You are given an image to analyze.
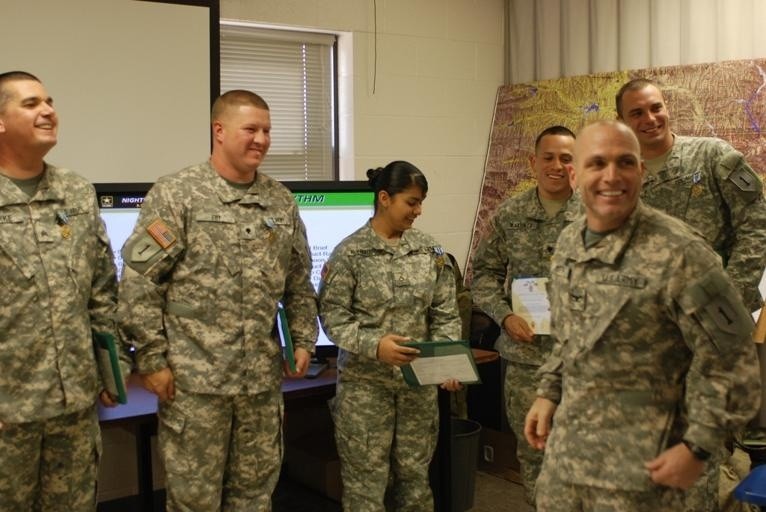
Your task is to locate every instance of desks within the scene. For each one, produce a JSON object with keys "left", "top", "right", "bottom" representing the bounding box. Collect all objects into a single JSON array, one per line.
[{"left": 96, "top": 345, "right": 501, "bottom": 511}]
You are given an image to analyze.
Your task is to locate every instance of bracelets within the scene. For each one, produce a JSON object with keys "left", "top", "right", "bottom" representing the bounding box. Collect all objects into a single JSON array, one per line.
[{"left": 683, "top": 440, "right": 710, "bottom": 463}]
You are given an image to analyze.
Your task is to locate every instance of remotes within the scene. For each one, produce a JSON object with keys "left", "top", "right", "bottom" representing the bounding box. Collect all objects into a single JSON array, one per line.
[{"left": 305, "top": 363, "right": 328, "bottom": 378}]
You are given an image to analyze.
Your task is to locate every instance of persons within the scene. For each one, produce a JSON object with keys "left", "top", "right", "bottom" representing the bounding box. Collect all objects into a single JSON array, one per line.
[
  {"left": 615, "top": 76, "right": 765, "bottom": 512},
  {"left": 0, "top": 70, "right": 133, "bottom": 512},
  {"left": 317, "top": 157, "right": 468, "bottom": 511},
  {"left": 114, "top": 89, "right": 319, "bottom": 512},
  {"left": 468, "top": 124, "right": 585, "bottom": 511},
  {"left": 522, "top": 116, "right": 766, "bottom": 512}
]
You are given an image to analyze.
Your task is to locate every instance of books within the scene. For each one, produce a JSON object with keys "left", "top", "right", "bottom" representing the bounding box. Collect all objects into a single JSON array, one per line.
[
  {"left": 90, "top": 330, "right": 127, "bottom": 406},
  {"left": 277, "top": 306, "right": 297, "bottom": 376},
  {"left": 397, "top": 340, "right": 483, "bottom": 392}
]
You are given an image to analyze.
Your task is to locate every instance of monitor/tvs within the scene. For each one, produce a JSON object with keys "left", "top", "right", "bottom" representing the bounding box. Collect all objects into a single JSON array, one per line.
[
  {"left": 277, "top": 181, "right": 375, "bottom": 369},
  {"left": 92, "top": 182, "right": 154, "bottom": 373}
]
